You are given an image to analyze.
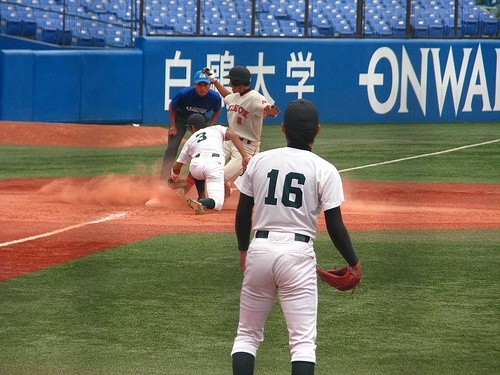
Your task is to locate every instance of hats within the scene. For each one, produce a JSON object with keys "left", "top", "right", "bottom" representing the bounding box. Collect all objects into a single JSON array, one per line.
[
  {"left": 284, "top": 98, "right": 318, "bottom": 129},
  {"left": 193, "top": 70, "right": 211, "bottom": 84},
  {"left": 223, "top": 66, "right": 251, "bottom": 83},
  {"left": 185, "top": 113, "right": 206, "bottom": 128}
]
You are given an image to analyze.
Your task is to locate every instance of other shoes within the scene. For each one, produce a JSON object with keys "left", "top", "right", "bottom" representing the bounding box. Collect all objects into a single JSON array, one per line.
[{"left": 186, "top": 198, "right": 204, "bottom": 214}]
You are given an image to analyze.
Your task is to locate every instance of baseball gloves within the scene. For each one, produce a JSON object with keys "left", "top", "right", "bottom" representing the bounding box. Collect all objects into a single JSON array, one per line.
[
  {"left": 318, "top": 266, "right": 360, "bottom": 291},
  {"left": 167, "top": 177, "right": 188, "bottom": 189}
]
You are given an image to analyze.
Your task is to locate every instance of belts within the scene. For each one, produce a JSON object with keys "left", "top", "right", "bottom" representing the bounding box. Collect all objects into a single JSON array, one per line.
[{"left": 255, "top": 230, "right": 311, "bottom": 243}]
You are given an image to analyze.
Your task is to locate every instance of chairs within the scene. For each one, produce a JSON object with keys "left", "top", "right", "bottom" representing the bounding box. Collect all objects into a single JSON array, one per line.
[{"left": 0, "top": 0, "right": 500, "bottom": 46}]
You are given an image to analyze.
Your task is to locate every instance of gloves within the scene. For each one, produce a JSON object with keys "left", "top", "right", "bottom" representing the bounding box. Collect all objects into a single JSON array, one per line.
[{"left": 202, "top": 67, "right": 217, "bottom": 83}]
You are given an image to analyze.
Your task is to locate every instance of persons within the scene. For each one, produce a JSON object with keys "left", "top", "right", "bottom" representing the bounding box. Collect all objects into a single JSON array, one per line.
[
  {"left": 230, "top": 97, "right": 362, "bottom": 375},
  {"left": 170, "top": 113, "right": 251, "bottom": 215},
  {"left": 175, "top": 66, "right": 280, "bottom": 205},
  {"left": 158, "top": 69, "right": 222, "bottom": 181}
]
